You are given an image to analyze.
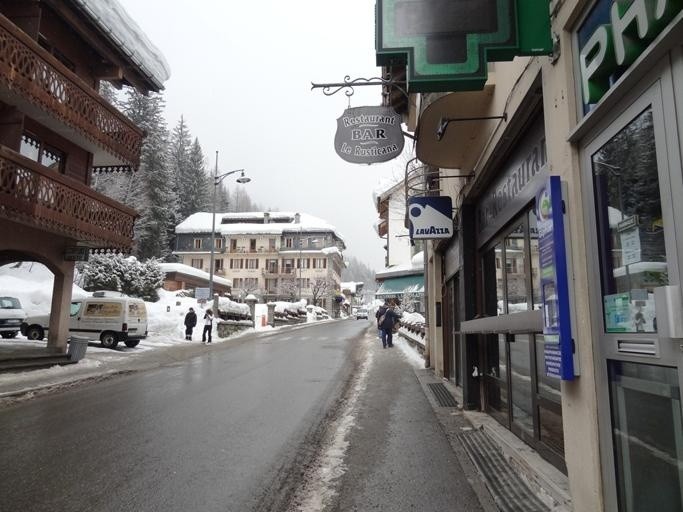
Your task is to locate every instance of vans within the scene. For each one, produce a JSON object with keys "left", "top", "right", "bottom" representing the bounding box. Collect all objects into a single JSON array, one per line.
[
  {"left": 20, "top": 294, "right": 148, "bottom": 349},
  {"left": 0, "top": 295, "right": 27, "bottom": 340}
]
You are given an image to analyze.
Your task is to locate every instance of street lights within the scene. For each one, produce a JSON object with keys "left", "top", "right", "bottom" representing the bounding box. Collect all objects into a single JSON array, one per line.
[{"left": 208, "top": 150, "right": 251, "bottom": 302}]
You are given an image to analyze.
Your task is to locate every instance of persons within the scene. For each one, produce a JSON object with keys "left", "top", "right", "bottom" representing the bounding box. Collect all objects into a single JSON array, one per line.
[
  {"left": 183, "top": 307, "right": 196, "bottom": 340},
  {"left": 201, "top": 308, "right": 214, "bottom": 345},
  {"left": 374, "top": 300, "right": 404, "bottom": 349}
]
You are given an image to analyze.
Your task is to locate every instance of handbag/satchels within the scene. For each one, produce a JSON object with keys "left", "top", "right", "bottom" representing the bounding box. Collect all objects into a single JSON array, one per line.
[{"left": 378, "top": 309, "right": 389, "bottom": 326}]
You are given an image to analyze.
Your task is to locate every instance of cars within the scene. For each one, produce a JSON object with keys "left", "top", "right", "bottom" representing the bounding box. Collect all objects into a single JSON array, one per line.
[{"left": 355, "top": 308, "right": 369, "bottom": 320}]
